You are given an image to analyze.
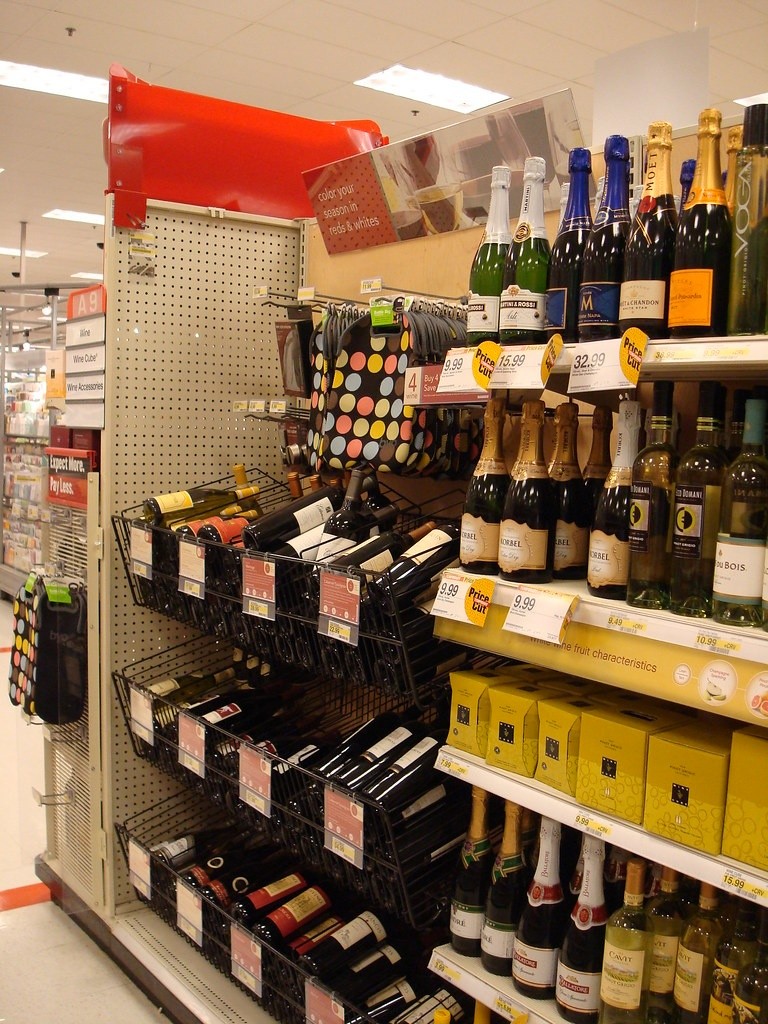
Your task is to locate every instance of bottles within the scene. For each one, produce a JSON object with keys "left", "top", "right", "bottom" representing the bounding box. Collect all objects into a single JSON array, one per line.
[
  {"left": 498, "top": 400, "right": 552, "bottom": 584},
  {"left": 133, "top": 465, "right": 473, "bottom": 695},
  {"left": 461, "top": 398, "right": 511, "bottom": 575},
  {"left": 543, "top": 148, "right": 593, "bottom": 344},
  {"left": 480, "top": 799, "right": 528, "bottom": 976},
  {"left": 136, "top": 817, "right": 471, "bottom": 1024},
  {"left": 558, "top": 103, "right": 768, "bottom": 337},
  {"left": 498, "top": 156, "right": 551, "bottom": 345},
  {"left": 512, "top": 808, "right": 768, "bottom": 1024},
  {"left": 547, "top": 399, "right": 768, "bottom": 632},
  {"left": 451, "top": 784, "right": 495, "bottom": 957},
  {"left": 467, "top": 165, "right": 513, "bottom": 348},
  {"left": 113, "top": 641, "right": 472, "bottom": 933}
]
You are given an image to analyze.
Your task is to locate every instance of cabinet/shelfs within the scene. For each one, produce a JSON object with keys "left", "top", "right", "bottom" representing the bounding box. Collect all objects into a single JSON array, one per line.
[{"left": 427, "top": 322, "right": 768, "bottom": 1024}]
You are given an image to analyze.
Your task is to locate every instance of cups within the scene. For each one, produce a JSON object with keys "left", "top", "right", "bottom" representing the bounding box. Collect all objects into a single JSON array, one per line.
[
  {"left": 372, "top": 131, "right": 463, "bottom": 242},
  {"left": 440, "top": 110, "right": 533, "bottom": 230},
  {"left": 543, "top": 91, "right": 584, "bottom": 176}
]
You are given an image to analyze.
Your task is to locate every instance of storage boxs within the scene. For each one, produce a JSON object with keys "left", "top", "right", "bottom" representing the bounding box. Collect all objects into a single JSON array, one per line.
[
  {"left": 445, "top": 665, "right": 768, "bottom": 875},
  {"left": 49, "top": 425, "right": 100, "bottom": 472}
]
